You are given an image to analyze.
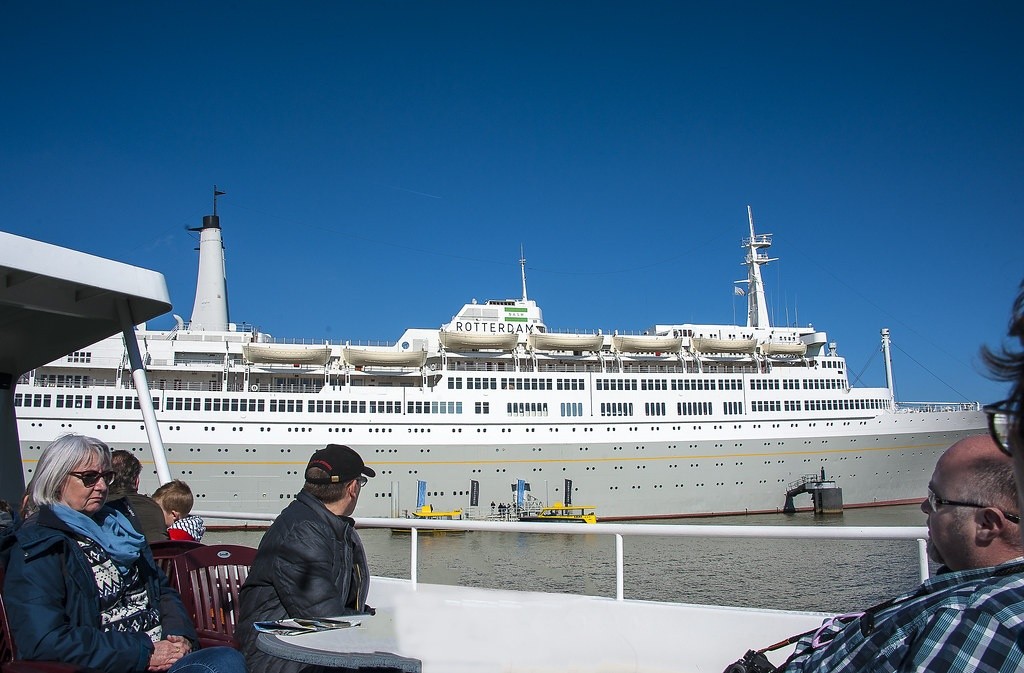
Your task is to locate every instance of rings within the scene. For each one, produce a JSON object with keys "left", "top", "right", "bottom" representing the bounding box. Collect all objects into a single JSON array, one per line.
[{"left": 175, "top": 647, "right": 180, "bottom": 653}]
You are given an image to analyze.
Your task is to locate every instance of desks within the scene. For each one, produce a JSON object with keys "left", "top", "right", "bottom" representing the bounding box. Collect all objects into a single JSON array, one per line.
[{"left": 255, "top": 608, "right": 423, "bottom": 673}]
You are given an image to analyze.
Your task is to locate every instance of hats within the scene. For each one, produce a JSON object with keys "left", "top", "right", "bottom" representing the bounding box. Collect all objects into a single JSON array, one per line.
[{"left": 305, "top": 444, "right": 376, "bottom": 484}]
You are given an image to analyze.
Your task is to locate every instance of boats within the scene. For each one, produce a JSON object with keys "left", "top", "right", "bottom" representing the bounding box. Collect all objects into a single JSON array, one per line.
[
  {"left": 613, "top": 332, "right": 684, "bottom": 353},
  {"left": 760, "top": 340, "right": 808, "bottom": 356},
  {"left": 389, "top": 478, "right": 463, "bottom": 535},
  {"left": 528, "top": 331, "right": 605, "bottom": 352},
  {"left": 343, "top": 346, "right": 430, "bottom": 368},
  {"left": 466, "top": 479, "right": 544, "bottom": 522},
  {"left": 692, "top": 336, "right": 758, "bottom": 355},
  {"left": 439, "top": 329, "right": 520, "bottom": 351},
  {"left": 517, "top": 479, "right": 599, "bottom": 524},
  {"left": 241, "top": 344, "right": 333, "bottom": 366}
]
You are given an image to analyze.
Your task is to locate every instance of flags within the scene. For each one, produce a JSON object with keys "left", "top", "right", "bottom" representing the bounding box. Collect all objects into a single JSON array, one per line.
[
  {"left": 517, "top": 480, "right": 525, "bottom": 507},
  {"left": 417, "top": 480, "right": 426, "bottom": 507},
  {"left": 564, "top": 478, "right": 572, "bottom": 504},
  {"left": 470, "top": 479, "right": 479, "bottom": 506}
]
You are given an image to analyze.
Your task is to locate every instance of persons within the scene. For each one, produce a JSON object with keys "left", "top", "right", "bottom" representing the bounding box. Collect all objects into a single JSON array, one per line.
[
  {"left": 775, "top": 433, "right": 1024, "bottom": 672},
  {"left": 1, "top": 433, "right": 249, "bottom": 673},
  {"left": 491, "top": 501, "right": 519, "bottom": 518},
  {"left": 982, "top": 278, "right": 1024, "bottom": 555},
  {"left": 235, "top": 442, "right": 396, "bottom": 673}
]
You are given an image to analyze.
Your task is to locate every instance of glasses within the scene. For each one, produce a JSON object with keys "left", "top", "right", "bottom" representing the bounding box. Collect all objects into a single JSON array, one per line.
[
  {"left": 926, "top": 484, "right": 1020, "bottom": 524},
  {"left": 345, "top": 476, "right": 369, "bottom": 488},
  {"left": 68, "top": 470, "right": 116, "bottom": 488},
  {"left": 982, "top": 399, "right": 1024, "bottom": 458}
]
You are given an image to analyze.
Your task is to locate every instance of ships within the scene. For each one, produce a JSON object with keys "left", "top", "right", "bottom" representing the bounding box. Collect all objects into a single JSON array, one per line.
[{"left": 14, "top": 206, "right": 1014, "bottom": 534}]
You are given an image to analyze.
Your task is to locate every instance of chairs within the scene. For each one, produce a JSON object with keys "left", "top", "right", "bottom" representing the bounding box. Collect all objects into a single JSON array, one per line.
[{"left": 0, "top": 539, "right": 258, "bottom": 673}]
